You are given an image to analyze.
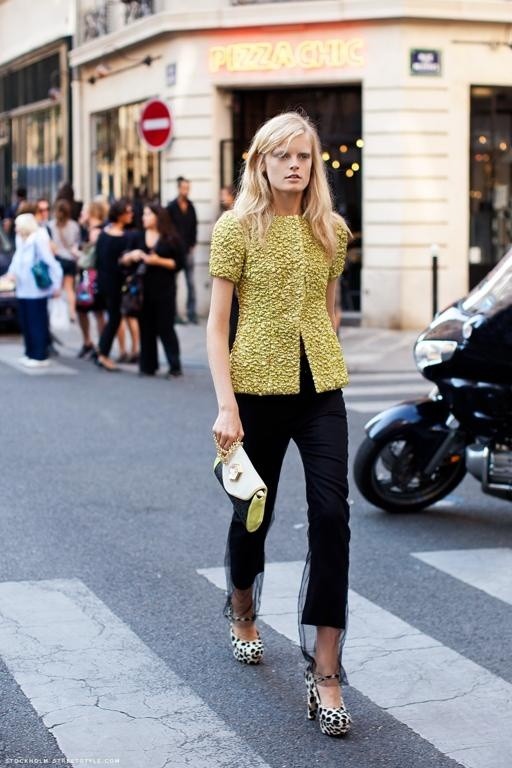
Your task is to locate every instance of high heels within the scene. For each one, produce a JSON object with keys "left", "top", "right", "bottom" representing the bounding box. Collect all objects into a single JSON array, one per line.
[
  {"left": 304, "top": 661, "right": 352, "bottom": 737},
  {"left": 224, "top": 592, "right": 264, "bottom": 664}
]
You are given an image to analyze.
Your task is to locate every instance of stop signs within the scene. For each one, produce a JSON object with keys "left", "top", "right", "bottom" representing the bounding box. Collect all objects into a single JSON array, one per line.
[{"left": 137, "top": 99, "right": 177, "bottom": 152}]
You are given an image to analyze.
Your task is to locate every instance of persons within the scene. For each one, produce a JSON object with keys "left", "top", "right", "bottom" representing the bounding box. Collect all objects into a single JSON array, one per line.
[
  {"left": 206, "top": 111, "right": 353, "bottom": 737},
  {"left": 219, "top": 186, "right": 234, "bottom": 211}
]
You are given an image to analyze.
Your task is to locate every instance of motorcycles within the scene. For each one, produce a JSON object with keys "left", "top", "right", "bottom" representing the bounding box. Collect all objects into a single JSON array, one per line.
[{"left": 351, "top": 238, "right": 512, "bottom": 519}]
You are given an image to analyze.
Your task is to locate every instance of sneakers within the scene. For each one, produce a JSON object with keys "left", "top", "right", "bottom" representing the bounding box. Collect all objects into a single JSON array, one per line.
[
  {"left": 77, "top": 343, "right": 181, "bottom": 379},
  {"left": 176, "top": 313, "right": 197, "bottom": 325},
  {"left": 19, "top": 355, "right": 52, "bottom": 368}
]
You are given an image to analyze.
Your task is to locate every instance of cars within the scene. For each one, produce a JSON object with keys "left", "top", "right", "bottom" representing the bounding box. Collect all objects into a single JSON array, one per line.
[{"left": 0, "top": 225, "right": 21, "bottom": 337}]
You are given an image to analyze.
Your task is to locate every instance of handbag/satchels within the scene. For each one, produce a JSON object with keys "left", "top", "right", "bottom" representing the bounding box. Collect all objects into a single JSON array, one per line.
[
  {"left": 76, "top": 243, "right": 97, "bottom": 270},
  {"left": 215, "top": 441, "right": 269, "bottom": 534},
  {"left": 121, "top": 275, "right": 144, "bottom": 311},
  {"left": 75, "top": 268, "right": 100, "bottom": 306},
  {"left": 31, "top": 260, "right": 51, "bottom": 289}
]
